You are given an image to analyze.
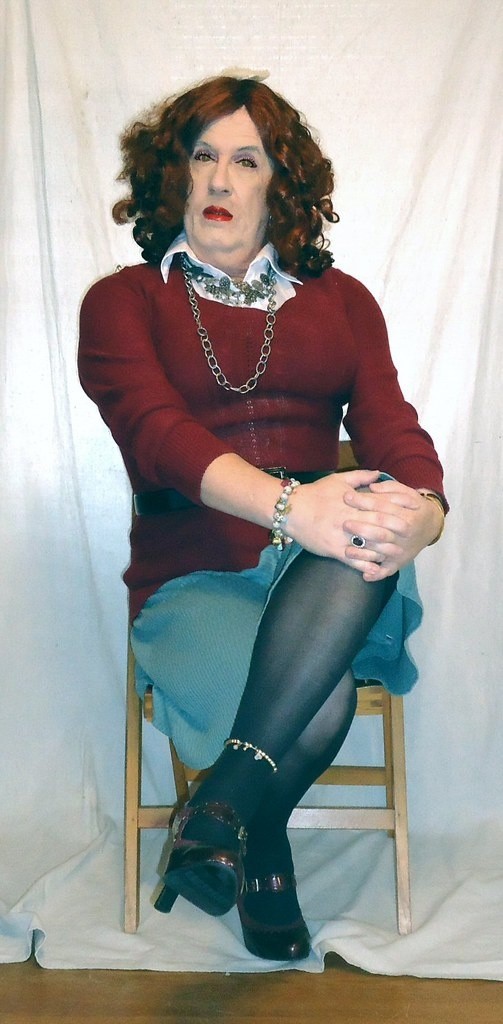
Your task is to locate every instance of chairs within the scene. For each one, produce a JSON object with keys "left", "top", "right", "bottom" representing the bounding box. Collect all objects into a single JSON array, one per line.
[{"left": 121, "top": 441, "right": 417, "bottom": 937}]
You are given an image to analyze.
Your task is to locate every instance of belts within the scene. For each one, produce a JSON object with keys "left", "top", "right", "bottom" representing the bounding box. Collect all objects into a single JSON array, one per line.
[{"left": 131, "top": 467, "right": 337, "bottom": 516}]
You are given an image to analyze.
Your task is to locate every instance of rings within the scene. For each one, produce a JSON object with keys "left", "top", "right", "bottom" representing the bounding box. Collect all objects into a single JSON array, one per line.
[{"left": 351, "top": 535, "right": 366, "bottom": 549}]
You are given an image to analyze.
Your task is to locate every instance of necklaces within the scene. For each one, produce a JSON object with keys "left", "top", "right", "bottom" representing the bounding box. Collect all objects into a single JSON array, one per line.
[{"left": 181, "top": 256, "right": 276, "bottom": 393}]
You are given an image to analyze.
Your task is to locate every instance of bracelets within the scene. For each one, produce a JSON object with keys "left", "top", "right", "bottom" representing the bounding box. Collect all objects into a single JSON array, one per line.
[
  {"left": 269, "top": 478, "right": 300, "bottom": 551},
  {"left": 420, "top": 493, "right": 445, "bottom": 545}
]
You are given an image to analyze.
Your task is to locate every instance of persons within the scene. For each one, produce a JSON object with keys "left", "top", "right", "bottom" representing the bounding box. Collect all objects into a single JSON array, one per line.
[{"left": 77, "top": 77, "right": 450, "bottom": 960}]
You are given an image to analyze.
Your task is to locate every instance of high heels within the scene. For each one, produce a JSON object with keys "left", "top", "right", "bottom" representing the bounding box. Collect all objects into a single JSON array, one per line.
[
  {"left": 154, "top": 800, "right": 246, "bottom": 917},
  {"left": 237, "top": 874, "right": 311, "bottom": 961}
]
